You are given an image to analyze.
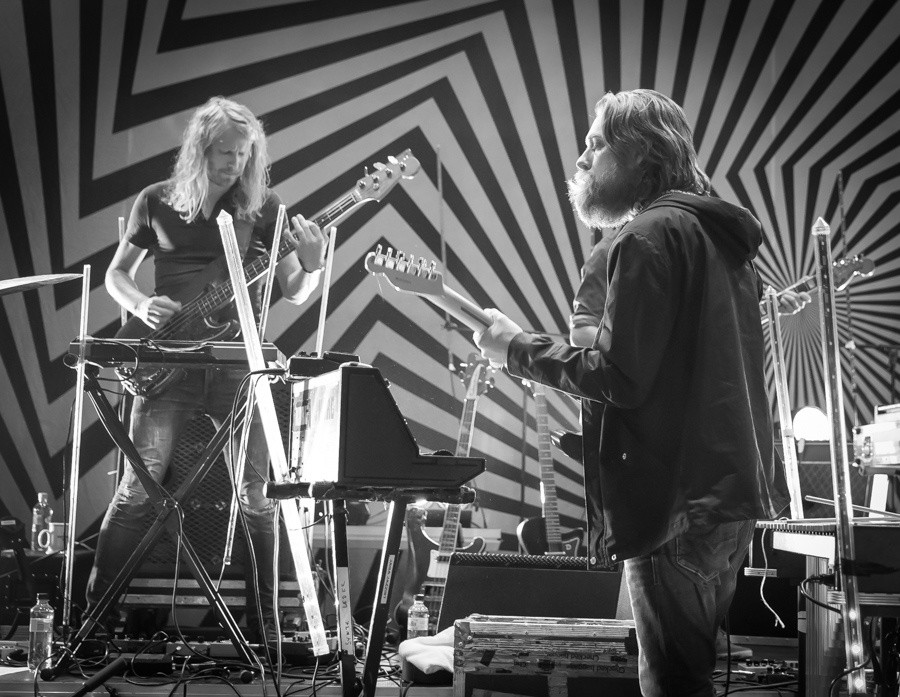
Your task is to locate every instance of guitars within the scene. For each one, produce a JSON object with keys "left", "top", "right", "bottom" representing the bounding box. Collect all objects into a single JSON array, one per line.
[
  {"left": 513, "top": 378, "right": 582, "bottom": 558},
  {"left": 109, "top": 149, "right": 422, "bottom": 397},
  {"left": 395, "top": 354, "right": 496, "bottom": 643},
  {"left": 365, "top": 238, "right": 496, "bottom": 337},
  {"left": 753, "top": 256, "right": 877, "bottom": 315}
]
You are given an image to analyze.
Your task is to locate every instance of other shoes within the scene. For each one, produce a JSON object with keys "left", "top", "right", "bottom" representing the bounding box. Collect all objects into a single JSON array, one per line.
[{"left": 715, "top": 633, "right": 753, "bottom": 658}]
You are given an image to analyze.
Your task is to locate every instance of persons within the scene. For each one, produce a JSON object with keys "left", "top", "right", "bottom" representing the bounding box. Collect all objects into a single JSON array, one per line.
[
  {"left": 83, "top": 97, "right": 329, "bottom": 656},
  {"left": 473, "top": 87, "right": 793, "bottom": 697}
]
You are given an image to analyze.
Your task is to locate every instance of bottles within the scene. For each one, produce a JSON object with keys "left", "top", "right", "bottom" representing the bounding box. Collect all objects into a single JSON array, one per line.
[
  {"left": 406, "top": 594, "right": 429, "bottom": 640},
  {"left": 31, "top": 491, "right": 53, "bottom": 552},
  {"left": 27, "top": 593, "right": 54, "bottom": 674}
]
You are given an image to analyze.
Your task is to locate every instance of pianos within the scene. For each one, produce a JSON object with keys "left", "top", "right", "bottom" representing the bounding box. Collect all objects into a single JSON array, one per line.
[{"left": 69, "top": 331, "right": 280, "bottom": 680}]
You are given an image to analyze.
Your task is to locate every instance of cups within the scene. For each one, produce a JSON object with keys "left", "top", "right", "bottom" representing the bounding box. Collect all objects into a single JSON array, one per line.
[{"left": 38, "top": 523, "right": 68, "bottom": 552}]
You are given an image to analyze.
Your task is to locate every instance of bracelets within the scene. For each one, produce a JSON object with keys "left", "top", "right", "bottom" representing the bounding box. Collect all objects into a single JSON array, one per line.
[{"left": 302, "top": 268, "right": 317, "bottom": 274}]
